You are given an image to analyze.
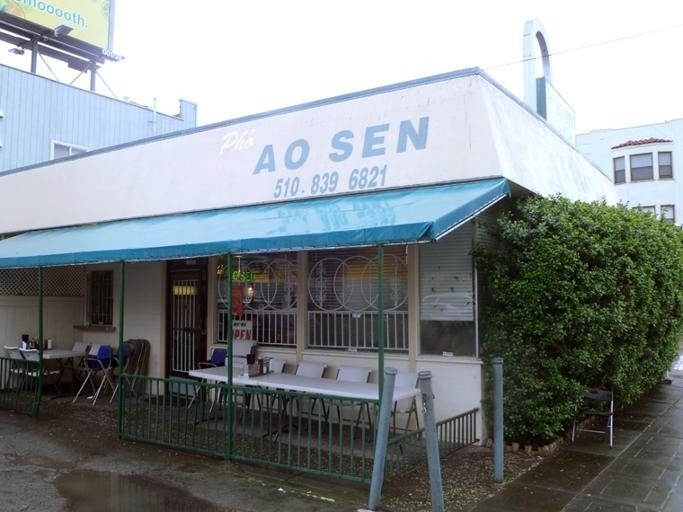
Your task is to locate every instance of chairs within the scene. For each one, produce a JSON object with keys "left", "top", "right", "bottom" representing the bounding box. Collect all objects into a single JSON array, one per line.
[
  {"left": 4, "top": 345, "right": 61, "bottom": 408},
  {"left": 186, "top": 348, "right": 420, "bottom": 449},
  {"left": 54, "top": 341, "right": 138, "bottom": 405}
]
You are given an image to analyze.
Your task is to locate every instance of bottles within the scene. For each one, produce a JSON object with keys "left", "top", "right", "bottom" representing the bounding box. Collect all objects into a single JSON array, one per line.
[{"left": 30, "top": 337, "right": 35, "bottom": 349}]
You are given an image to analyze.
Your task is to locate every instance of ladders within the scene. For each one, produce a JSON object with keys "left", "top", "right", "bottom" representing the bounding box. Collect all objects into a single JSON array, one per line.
[{"left": 570, "top": 383, "right": 613, "bottom": 448}]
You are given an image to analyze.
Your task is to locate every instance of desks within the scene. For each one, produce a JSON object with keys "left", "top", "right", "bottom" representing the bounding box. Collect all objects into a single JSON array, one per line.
[{"left": 25, "top": 349, "right": 87, "bottom": 400}]
